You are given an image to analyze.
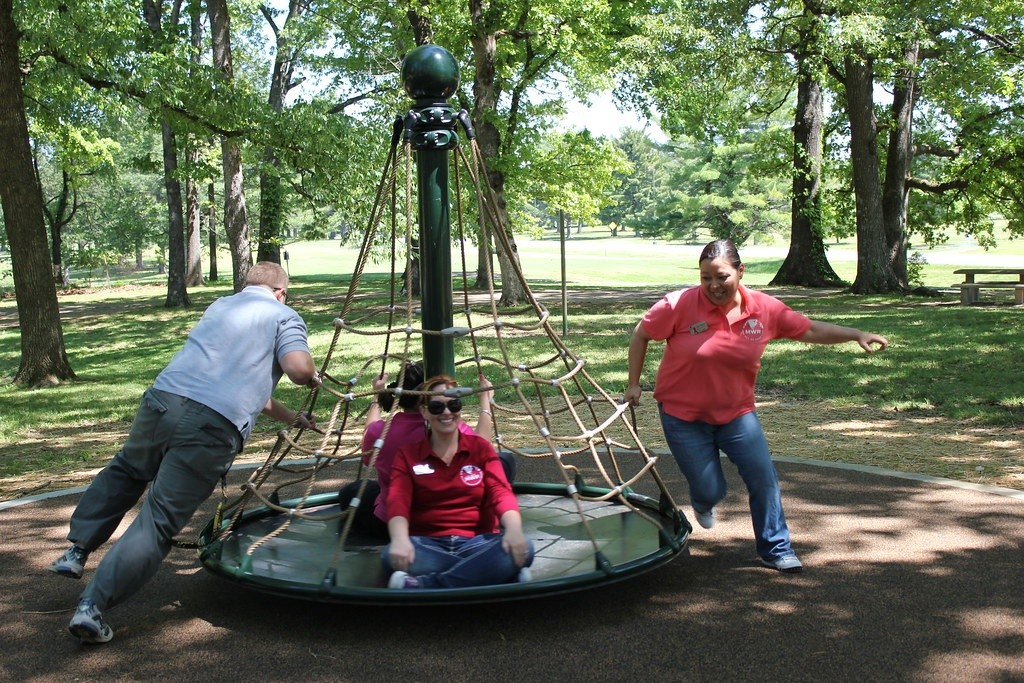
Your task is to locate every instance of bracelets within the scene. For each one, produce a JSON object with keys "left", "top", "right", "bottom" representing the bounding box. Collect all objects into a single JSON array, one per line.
[{"left": 479, "top": 410, "right": 492, "bottom": 416}]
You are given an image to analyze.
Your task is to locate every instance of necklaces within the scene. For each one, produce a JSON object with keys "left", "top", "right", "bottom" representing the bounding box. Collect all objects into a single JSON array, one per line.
[{"left": 433, "top": 447, "right": 456, "bottom": 467}]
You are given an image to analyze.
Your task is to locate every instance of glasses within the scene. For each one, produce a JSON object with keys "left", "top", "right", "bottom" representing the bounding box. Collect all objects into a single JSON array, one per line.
[
  {"left": 426, "top": 398, "right": 463, "bottom": 416},
  {"left": 273, "top": 286, "right": 289, "bottom": 304}
]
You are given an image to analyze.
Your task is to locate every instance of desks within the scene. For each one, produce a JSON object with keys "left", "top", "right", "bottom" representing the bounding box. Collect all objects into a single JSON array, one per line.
[{"left": 953, "top": 269, "right": 1024, "bottom": 303}]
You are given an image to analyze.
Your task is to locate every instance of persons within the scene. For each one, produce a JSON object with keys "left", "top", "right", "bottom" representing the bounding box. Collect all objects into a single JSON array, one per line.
[
  {"left": 624, "top": 237, "right": 887, "bottom": 573},
  {"left": 48, "top": 260, "right": 322, "bottom": 642},
  {"left": 339, "top": 362, "right": 517, "bottom": 536},
  {"left": 382, "top": 377, "right": 535, "bottom": 589}
]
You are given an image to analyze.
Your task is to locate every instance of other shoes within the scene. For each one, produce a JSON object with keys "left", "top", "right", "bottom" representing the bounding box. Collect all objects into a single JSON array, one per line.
[
  {"left": 387, "top": 570, "right": 420, "bottom": 590},
  {"left": 510, "top": 568, "right": 534, "bottom": 582}
]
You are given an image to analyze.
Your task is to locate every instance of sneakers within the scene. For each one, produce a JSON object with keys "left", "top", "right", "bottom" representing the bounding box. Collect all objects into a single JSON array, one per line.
[
  {"left": 761, "top": 554, "right": 803, "bottom": 573},
  {"left": 68, "top": 599, "right": 114, "bottom": 644},
  {"left": 48, "top": 545, "right": 89, "bottom": 579},
  {"left": 693, "top": 506, "right": 716, "bottom": 529}
]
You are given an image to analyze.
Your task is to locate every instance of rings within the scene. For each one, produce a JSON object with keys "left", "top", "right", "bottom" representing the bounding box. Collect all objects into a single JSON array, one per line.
[{"left": 523, "top": 550, "right": 528, "bottom": 554}]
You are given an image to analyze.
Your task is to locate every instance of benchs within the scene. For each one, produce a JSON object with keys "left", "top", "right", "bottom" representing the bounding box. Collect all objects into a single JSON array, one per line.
[{"left": 950, "top": 281, "right": 1024, "bottom": 306}]
